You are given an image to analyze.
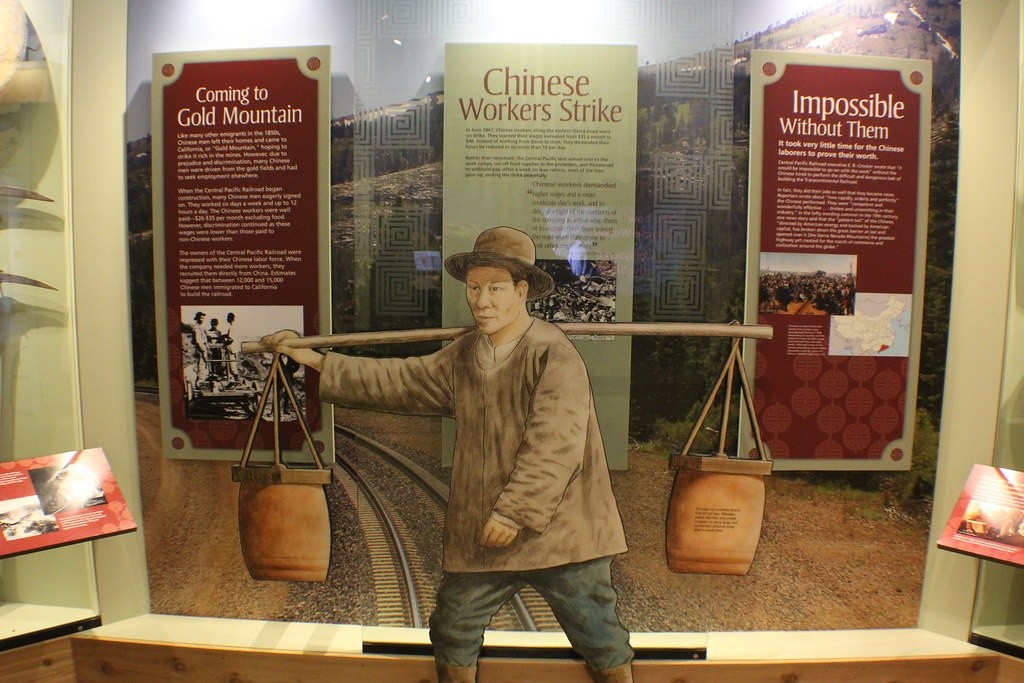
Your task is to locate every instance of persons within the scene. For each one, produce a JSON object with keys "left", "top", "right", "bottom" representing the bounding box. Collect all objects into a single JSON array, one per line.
[{"left": 569, "top": 236, "right": 588, "bottom": 277}]
